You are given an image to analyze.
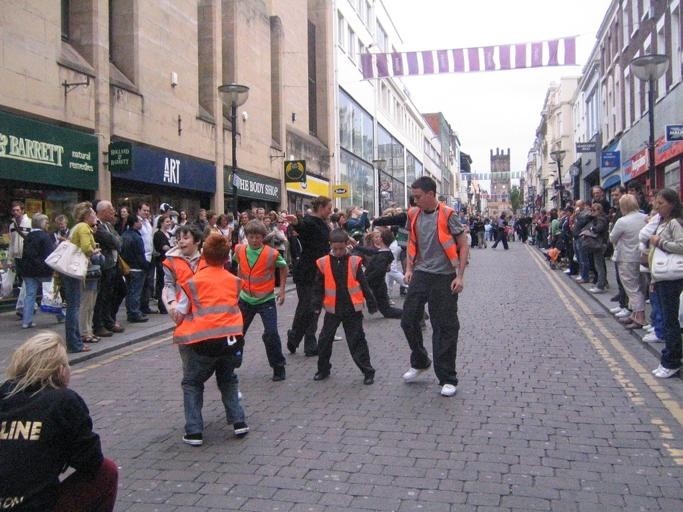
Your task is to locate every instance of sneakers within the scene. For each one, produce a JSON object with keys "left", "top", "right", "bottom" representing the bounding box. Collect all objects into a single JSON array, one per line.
[
  {"left": 233, "top": 422, "right": 250, "bottom": 436},
  {"left": 306, "top": 350, "right": 318, "bottom": 357},
  {"left": 608, "top": 306, "right": 663, "bottom": 343},
  {"left": 561, "top": 267, "right": 608, "bottom": 296},
  {"left": 287, "top": 329, "right": 296, "bottom": 353},
  {"left": 271, "top": 364, "right": 286, "bottom": 381},
  {"left": 181, "top": 432, "right": 204, "bottom": 446},
  {"left": 313, "top": 369, "right": 331, "bottom": 380},
  {"left": 439, "top": 380, "right": 458, "bottom": 398},
  {"left": 402, "top": 360, "right": 433, "bottom": 384},
  {"left": 651, "top": 362, "right": 664, "bottom": 376},
  {"left": 363, "top": 367, "right": 374, "bottom": 385},
  {"left": 654, "top": 365, "right": 682, "bottom": 379}
]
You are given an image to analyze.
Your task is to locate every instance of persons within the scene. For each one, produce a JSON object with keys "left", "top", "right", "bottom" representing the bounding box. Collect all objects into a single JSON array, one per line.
[
  {"left": 231, "top": 219, "right": 287, "bottom": 381},
  {"left": 0, "top": 330, "right": 119, "bottom": 511},
  {"left": 370, "top": 176, "right": 469, "bottom": 397},
  {"left": 284, "top": 195, "right": 331, "bottom": 354},
  {"left": 56, "top": 201, "right": 102, "bottom": 354},
  {"left": 170, "top": 233, "right": 249, "bottom": 446},
  {"left": 287, "top": 163, "right": 302, "bottom": 178},
  {"left": 309, "top": 229, "right": 374, "bottom": 386},
  {"left": 649, "top": 188, "right": 683, "bottom": 377},
  {"left": 456, "top": 204, "right": 515, "bottom": 250},
  {"left": 329, "top": 203, "right": 409, "bottom": 304},
  {"left": 513, "top": 181, "right": 680, "bottom": 355},
  {"left": 9, "top": 199, "right": 285, "bottom": 326}
]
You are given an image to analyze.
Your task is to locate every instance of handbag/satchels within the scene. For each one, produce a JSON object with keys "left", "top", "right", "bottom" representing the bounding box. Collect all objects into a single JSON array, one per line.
[
  {"left": 44, "top": 222, "right": 90, "bottom": 281},
  {"left": 650, "top": 242, "right": 683, "bottom": 284},
  {"left": 117, "top": 252, "right": 131, "bottom": 276},
  {"left": 575, "top": 224, "right": 607, "bottom": 252}
]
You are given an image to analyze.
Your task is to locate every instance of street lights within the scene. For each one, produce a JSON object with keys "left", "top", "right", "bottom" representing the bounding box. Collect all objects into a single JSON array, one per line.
[
  {"left": 370, "top": 158, "right": 389, "bottom": 216},
  {"left": 456, "top": 197, "right": 463, "bottom": 214},
  {"left": 628, "top": 54, "right": 670, "bottom": 199},
  {"left": 218, "top": 79, "right": 252, "bottom": 230},
  {"left": 547, "top": 148, "right": 568, "bottom": 210},
  {"left": 539, "top": 176, "right": 549, "bottom": 208}
]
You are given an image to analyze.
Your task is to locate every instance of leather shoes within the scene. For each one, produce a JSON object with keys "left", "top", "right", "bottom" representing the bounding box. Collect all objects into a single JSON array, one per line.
[{"left": 67, "top": 306, "right": 159, "bottom": 354}]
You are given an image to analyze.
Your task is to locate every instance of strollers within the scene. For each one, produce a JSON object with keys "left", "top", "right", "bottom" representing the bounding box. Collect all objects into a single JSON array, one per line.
[{"left": 544, "top": 241, "right": 572, "bottom": 272}]
